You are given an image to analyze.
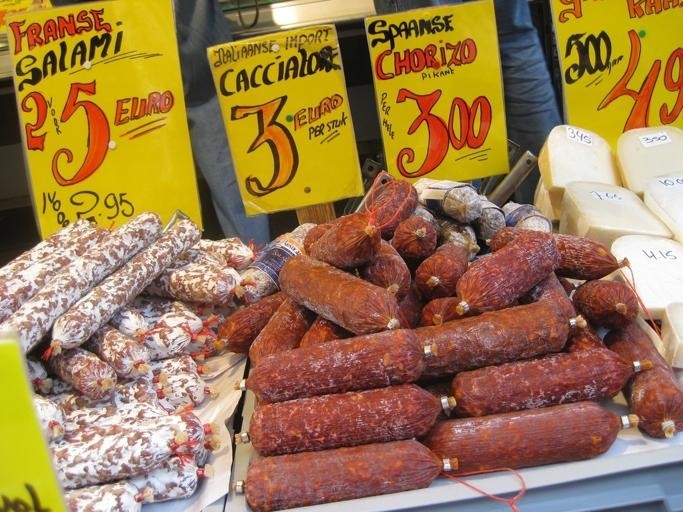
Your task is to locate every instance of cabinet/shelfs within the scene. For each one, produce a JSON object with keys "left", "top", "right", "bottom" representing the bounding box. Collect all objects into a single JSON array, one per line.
[{"left": 215, "top": 223, "right": 681, "bottom": 509}]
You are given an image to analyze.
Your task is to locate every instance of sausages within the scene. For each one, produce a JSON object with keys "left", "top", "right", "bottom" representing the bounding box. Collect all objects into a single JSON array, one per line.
[{"left": 0, "top": 178, "right": 683, "bottom": 512}]
